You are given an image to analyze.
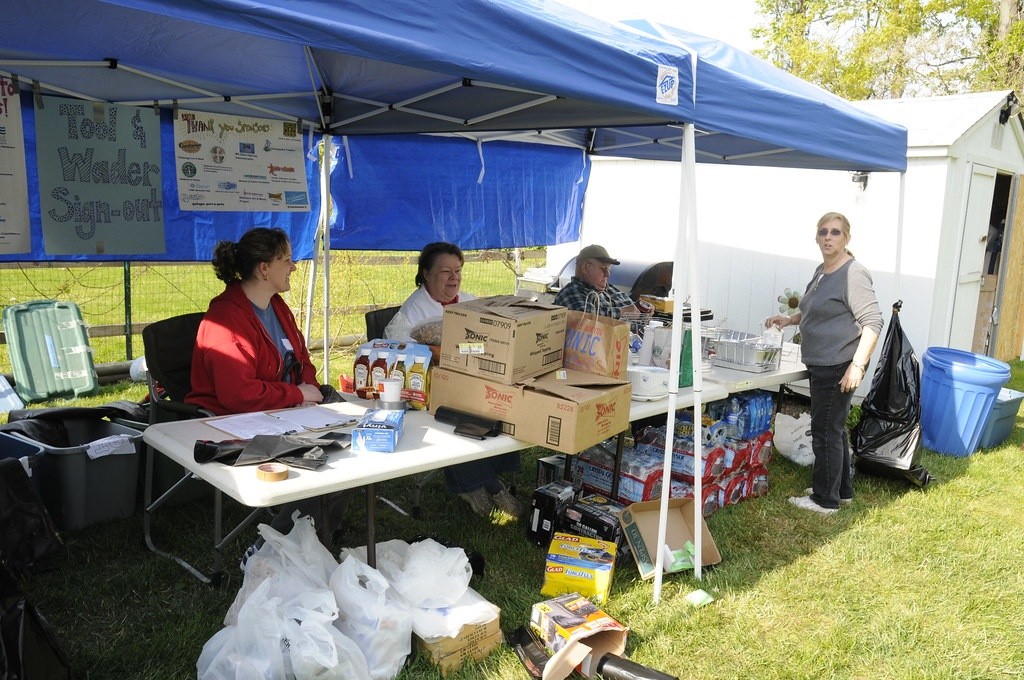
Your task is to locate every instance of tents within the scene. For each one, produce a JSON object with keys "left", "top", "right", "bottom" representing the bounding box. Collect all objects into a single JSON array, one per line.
[{"left": 0, "top": 0, "right": 907, "bottom": 604}]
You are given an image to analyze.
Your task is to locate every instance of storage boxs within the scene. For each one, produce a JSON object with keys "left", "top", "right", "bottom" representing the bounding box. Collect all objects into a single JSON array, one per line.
[
  {"left": 531, "top": 593, "right": 629, "bottom": 680},
  {"left": 0, "top": 431, "right": 46, "bottom": 503},
  {"left": 10, "top": 420, "right": 144, "bottom": 533},
  {"left": 438, "top": 295, "right": 568, "bottom": 386},
  {"left": 416, "top": 617, "right": 505, "bottom": 679},
  {"left": 619, "top": 499, "right": 720, "bottom": 581},
  {"left": 541, "top": 534, "right": 618, "bottom": 602},
  {"left": 431, "top": 365, "right": 632, "bottom": 455},
  {"left": 530, "top": 455, "right": 634, "bottom": 563},
  {"left": 979, "top": 387, "right": 1024, "bottom": 448},
  {"left": 351, "top": 409, "right": 405, "bottom": 452},
  {"left": 110, "top": 412, "right": 204, "bottom": 505}
]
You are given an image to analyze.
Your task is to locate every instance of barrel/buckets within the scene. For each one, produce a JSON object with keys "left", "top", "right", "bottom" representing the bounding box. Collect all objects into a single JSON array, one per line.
[{"left": 919, "top": 347, "right": 1011, "bottom": 458}]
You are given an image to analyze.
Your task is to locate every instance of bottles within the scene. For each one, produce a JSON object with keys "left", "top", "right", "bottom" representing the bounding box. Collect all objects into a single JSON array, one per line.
[
  {"left": 353, "top": 349, "right": 425, "bottom": 396},
  {"left": 709, "top": 393, "right": 774, "bottom": 442},
  {"left": 627, "top": 330, "right": 643, "bottom": 352}
]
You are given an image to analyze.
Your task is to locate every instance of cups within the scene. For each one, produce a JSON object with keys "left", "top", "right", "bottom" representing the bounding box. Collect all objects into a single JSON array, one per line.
[
  {"left": 701, "top": 334, "right": 720, "bottom": 373},
  {"left": 378, "top": 379, "right": 403, "bottom": 402}
]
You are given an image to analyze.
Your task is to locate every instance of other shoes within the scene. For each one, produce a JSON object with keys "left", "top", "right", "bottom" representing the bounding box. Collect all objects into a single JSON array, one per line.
[{"left": 239, "top": 544, "right": 256, "bottom": 575}]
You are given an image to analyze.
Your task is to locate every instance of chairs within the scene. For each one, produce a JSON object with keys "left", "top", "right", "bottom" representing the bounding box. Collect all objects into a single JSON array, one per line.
[
  {"left": 363, "top": 307, "right": 441, "bottom": 515},
  {"left": 141, "top": 311, "right": 263, "bottom": 589}
]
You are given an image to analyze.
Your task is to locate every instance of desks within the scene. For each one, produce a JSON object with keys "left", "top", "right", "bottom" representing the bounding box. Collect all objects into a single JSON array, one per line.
[{"left": 143, "top": 338, "right": 808, "bottom": 571}]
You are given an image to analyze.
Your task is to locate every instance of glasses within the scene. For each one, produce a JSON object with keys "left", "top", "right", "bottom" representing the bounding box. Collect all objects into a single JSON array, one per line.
[
  {"left": 584, "top": 261, "right": 609, "bottom": 275},
  {"left": 818, "top": 227, "right": 841, "bottom": 236}
]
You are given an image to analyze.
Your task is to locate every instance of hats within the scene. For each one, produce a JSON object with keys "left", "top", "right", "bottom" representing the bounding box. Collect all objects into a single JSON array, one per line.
[{"left": 576, "top": 244, "right": 620, "bottom": 265}]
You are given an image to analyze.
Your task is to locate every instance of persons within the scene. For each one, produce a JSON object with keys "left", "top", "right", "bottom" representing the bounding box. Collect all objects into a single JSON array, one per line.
[
  {"left": 184, "top": 227, "right": 348, "bottom": 569},
  {"left": 385, "top": 243, "right": 525, "bottom": 516},
  {"left": 764, "top": 212, "right": 884, "bottom": 514},
  {"left": 554, "top": 245, "right": 641, "bottom": 330}
]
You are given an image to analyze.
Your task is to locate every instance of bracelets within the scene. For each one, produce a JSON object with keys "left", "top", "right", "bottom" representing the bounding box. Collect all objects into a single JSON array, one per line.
[{"left": 851, "top": 363, "right": 865, "bottom": 373}]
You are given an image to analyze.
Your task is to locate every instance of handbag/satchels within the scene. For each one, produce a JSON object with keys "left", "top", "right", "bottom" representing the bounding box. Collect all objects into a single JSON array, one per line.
[{"left": 563, "top": 291, "right": 631, "bottom": 381}]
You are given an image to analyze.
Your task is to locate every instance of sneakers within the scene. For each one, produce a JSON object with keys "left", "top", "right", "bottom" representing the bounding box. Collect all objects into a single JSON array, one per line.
[
  {"left": 787, "top": 496, "right": 841, "bottom": 515},
  {"left": 804, "top": 487, "right": 853, "bottom": 504},
  {"left": 457, "top": 487, "right": 494, "bottom": 518},
  {"left": 487, "top": 477, "right": 525, "bottom": 518}
]
